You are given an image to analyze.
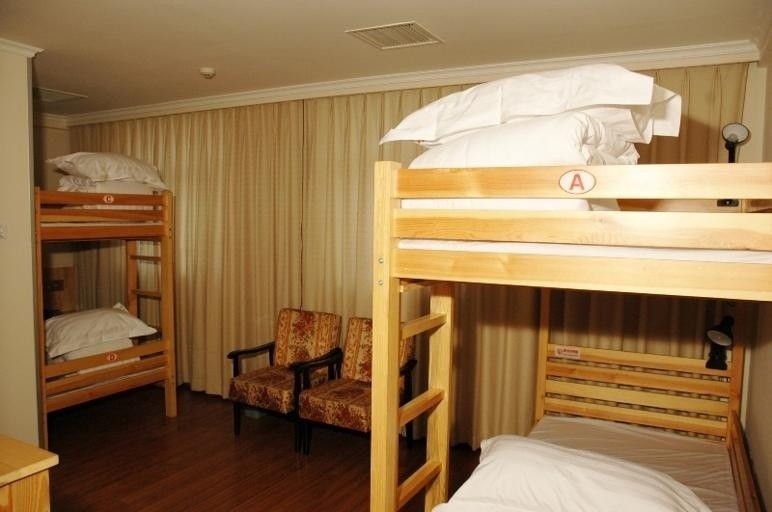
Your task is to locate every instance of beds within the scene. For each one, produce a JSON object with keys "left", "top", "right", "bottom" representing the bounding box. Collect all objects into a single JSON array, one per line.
[
  {"left": 365, "top": 157, "right": 772, "bottom": 511},
  {"left": 31, "top": 183, "right": 183, "bottom": 451}
]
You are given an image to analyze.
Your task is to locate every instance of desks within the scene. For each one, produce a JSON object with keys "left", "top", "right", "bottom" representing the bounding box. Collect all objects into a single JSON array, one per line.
[{"left": 0, "top": 433, "right": 63, "bottom": 512}]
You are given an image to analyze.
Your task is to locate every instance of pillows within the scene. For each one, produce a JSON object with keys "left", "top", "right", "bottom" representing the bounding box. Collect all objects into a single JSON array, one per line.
[
  {"left": 367, "top": 64, "right": 683, "bottom": 146},
  {"left": 44, "top": 151, "right": 175, "bottom": 194},
  {"left": 430, "top": 432, "right": 715, "bottom": 511},
  {"left": 39, "top": 300, "right": 163, "bottom": 361}
]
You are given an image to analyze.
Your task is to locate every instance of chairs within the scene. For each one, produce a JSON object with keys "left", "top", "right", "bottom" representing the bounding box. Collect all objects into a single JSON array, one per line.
[
  {"left": 299, "top": 315, "right": 418, "bottom": 456},
  {"left": 223, "top": 306, "right": 345, "bottom": 453}
]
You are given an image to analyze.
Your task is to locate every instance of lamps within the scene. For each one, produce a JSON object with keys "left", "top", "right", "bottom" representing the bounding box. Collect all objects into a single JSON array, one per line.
[
  {"left": 717, "top": 120, "right": 753, "bottom": 207},
  {"left": 703, "top": 313, "right": 736, "bottom": 374}
]
